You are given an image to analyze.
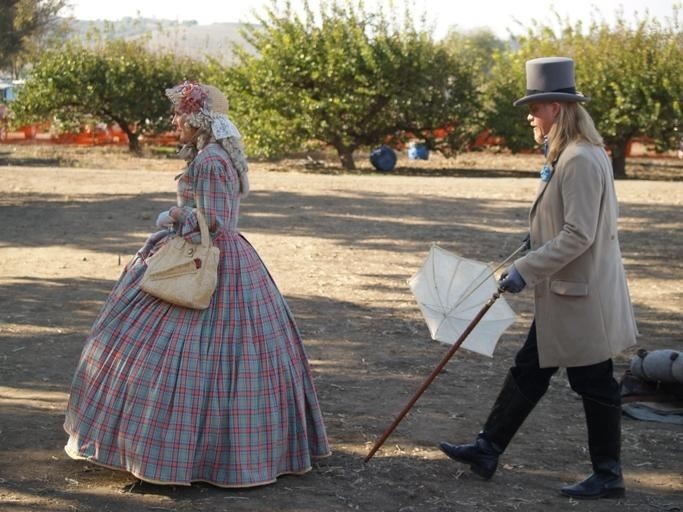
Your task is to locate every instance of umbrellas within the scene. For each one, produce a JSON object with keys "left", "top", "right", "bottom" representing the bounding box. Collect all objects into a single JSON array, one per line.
[{"left": 407, "top": 241, "right": 527, "bottom": 359}]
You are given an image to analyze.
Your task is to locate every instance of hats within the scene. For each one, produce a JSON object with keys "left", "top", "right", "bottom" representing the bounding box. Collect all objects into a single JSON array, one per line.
[
  {"left": 513, "top": 56, "right": 589, "bottom": 106},
  {"left": 165, "top": 79, "right": 229, "bottom": 121}
]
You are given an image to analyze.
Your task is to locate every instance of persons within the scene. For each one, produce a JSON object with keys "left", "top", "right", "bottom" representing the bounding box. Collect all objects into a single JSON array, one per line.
[
  {"left": 62, "top": 84, "right": 331, "bottom": 489},
  {"left": 440, "top": 58, "right": 638, "bottom": 497}
]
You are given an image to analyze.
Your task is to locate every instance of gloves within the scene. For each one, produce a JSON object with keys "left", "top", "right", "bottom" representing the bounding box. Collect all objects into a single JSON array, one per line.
[
  {"left": 497, "top": 264, "right": 526, "bottom": 294},
  {"left": 156, "top": 206, "right": 178, "bottom": 230}
]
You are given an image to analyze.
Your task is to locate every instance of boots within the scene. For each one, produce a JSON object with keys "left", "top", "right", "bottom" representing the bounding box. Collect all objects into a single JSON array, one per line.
[
  {"left": 438, "top": 370, "right": 535, "bottom": 479},
  {"left": 560, "top": 381, "right": 626, "bottom": 500}
]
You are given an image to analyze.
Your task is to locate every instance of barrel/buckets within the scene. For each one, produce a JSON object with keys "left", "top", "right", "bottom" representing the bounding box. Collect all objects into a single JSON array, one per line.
[
  {"left": 370, "top": 144, "right": 397, "bottom": 170},
  {"left": 409, "top": 141, "right": 429, "bottom": 159}
]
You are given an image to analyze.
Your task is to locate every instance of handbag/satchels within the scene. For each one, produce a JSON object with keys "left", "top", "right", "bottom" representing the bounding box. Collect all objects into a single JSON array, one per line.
[{"left": 139, "top": 237, "right": 220, "bottom": 311}]
